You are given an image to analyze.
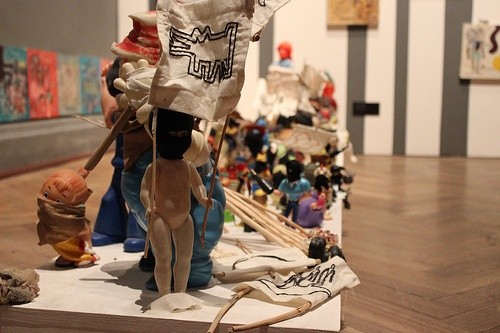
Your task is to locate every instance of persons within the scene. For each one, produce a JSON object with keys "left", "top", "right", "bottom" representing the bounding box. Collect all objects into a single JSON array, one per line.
[
  {"left": 89, "top": 10, "right": 353, "bottom": 295},
  {"left": 35, "top": 170, "right": 97, "bottom": 269}
]
